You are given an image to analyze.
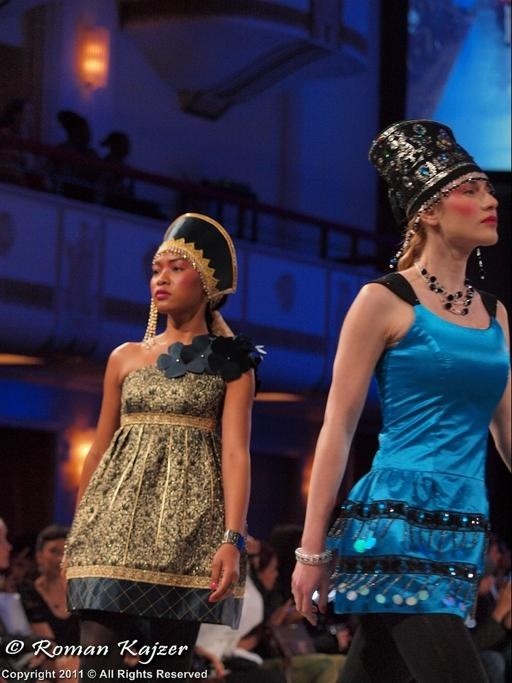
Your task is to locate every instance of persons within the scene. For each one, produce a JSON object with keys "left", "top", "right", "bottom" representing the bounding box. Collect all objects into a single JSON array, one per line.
[
  {"left": 60, "top": 212, "right": 262, "bottom": 683},
  {"left": 101, "top": 132, "right": 169, "bottom": 221},
  {"left": 52, "top": 111, "right": 133, "bottom": 213},
  {"left": 289, "top": 119, "right": 512, "bottom": 683},
  {"left": 0, "top": 104, "right": 54, "bottom": 193}
]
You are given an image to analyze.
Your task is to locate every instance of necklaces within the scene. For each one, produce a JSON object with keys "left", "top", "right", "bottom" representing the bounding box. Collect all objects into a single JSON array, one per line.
[{"left": 414, "top": 262, "right": 474, "bottom": 316}]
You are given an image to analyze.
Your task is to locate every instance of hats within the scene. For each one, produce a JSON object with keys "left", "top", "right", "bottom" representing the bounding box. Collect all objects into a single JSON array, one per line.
[
  {"left": 152, "top": 211, "right": 238, "bottom": 300},
  {"left": 368, "top": 118, "right": 491, "bottom": 219}
]
